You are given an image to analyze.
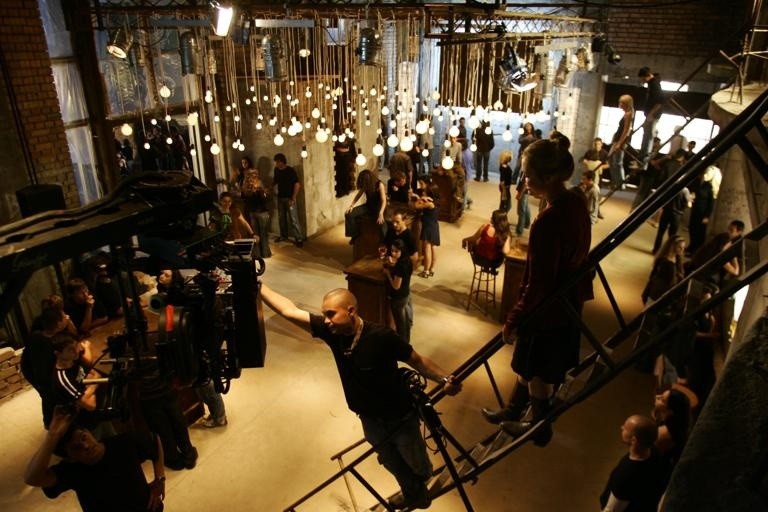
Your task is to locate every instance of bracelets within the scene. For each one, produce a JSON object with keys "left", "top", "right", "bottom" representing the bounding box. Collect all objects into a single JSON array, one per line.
[{"left": 441, "top": 375, "right": 451, "bottom": 384}]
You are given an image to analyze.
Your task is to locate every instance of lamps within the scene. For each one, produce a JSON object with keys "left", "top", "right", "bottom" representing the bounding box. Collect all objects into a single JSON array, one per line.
[
  {"left": 108, "top": 1, "right": 574, "bottom": 170},
  {"left": 493, "top": 36, "right": 537, "bottom": 95},
  {"left": 355, "top": 28, "right": 379, "bottom": 67},
  {"left": 591, "top": 24, "right": 621, "bottom": 65},
  {"left": 106, "top": 28, "right": 133, "bottom": 59}
]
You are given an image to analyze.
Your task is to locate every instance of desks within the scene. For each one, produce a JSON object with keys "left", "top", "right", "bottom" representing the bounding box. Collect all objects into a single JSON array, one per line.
[
  {"left": 347, "top": 205, "right": 383, "bottom": 261},
  {"left": 343, "top": 254, "right": 396, "bottom": 330},
  {"left": 501, "top": 225, "right": 530, "bottom": 322}
]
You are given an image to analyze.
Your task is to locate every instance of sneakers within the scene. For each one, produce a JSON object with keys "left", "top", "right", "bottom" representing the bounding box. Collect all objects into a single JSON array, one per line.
[
  {"left": 199, "top": 416, "right": 227, "bottom": 427},
  {"left": 275, "top": 236, "right": 286, "bottom": 242},
  {"left": 387, "top": 494, "right": 429, "bottom": 509},
  {"left": 296, "top": 240, "right": 304, "bottom": 247}
]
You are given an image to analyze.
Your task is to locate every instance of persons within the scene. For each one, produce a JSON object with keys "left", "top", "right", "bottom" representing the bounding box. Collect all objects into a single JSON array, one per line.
[
  {"left": 607, "top": 95, "right": 635, "bottom": 190},
  {"left": 629, "top": 65, "right": 665, "bottom": 171},
  {"left": 17, "top": 154, "right": 301, "bottom": 512},
  {"left": 257, "top": 279, "right": 462, "bottom": 510},
  {"left": 499, "top": 123, "right": 612, "bottom": 234},
  {"left": 480, "top": 131, "right": 592, "bottom": 448},
  {"left": 349, "top": 118, "right": 494, "bottom": 345},
  {"left": 599, "top": 125, "right": 743, "bottom": 512}
]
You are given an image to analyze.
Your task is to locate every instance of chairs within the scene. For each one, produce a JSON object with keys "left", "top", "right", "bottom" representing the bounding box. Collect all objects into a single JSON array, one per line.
[
  {"left": 430, "top": 174, "right": 462, "bottom": 223},
  {"left": 465, "top": 239, "right": 504, "bottom": 316}
]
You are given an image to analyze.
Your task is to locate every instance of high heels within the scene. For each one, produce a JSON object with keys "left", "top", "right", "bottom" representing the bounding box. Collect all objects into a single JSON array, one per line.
[{"left": 418, "top": 270, "right": 434, "bottom": 278}]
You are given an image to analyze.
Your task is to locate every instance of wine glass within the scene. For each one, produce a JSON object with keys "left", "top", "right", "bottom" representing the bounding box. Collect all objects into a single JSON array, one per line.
[{"left": 378, "top": 247, "right": 387, "bottom": 259}]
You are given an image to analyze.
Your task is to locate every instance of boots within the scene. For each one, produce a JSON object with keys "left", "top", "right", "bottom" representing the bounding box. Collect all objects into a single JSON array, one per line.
[
  {"left": 482, "top": 376, "right": 529, "bottom": 424},
  {"left": 499, "top": 392, "right": 553, "bottom": 447}
]
